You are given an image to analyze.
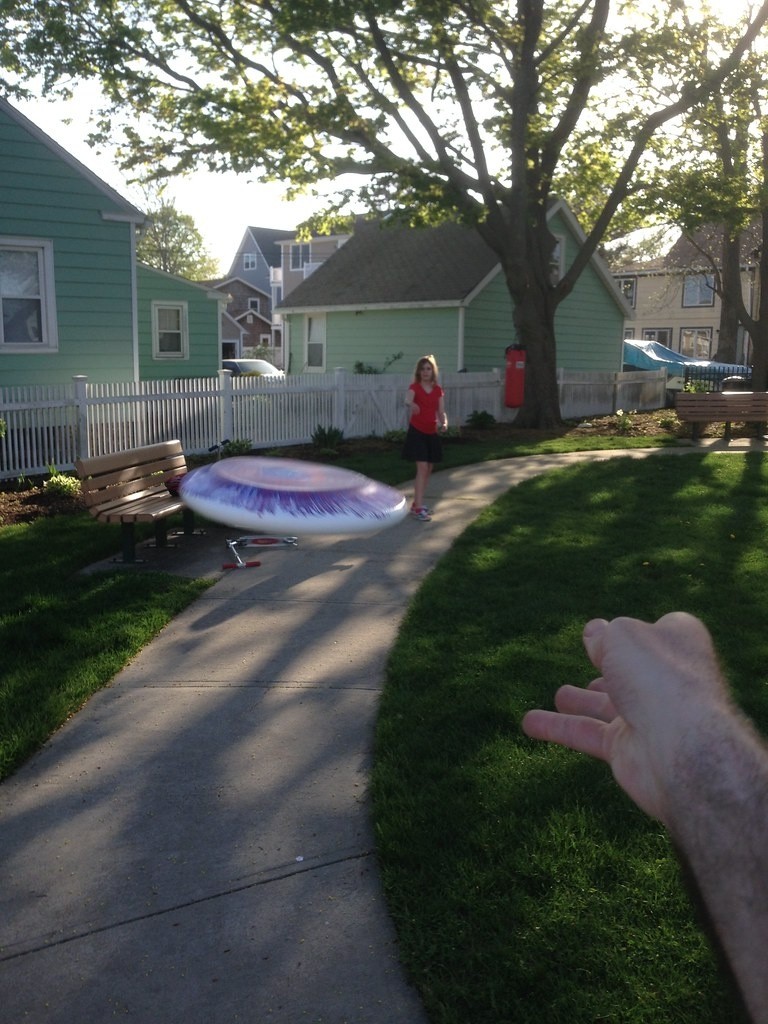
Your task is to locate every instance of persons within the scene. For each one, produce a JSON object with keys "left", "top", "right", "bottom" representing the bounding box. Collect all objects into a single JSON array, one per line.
[
  {"left": 521, "top": 609, "right": 767, "bottom": 1023},
  {"left": 403, "top": 355, "right": 448, "bottom": 519}
]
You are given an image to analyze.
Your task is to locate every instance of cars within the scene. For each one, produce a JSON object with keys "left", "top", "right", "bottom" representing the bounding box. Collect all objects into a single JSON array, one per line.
[{"left": 222, "top": 358, "right": 284, "bottom": 379}]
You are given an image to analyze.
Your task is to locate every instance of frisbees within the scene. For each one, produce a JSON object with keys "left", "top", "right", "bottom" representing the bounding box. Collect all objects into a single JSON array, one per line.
[{"left": 176, "top": 459, "right": 414, "bottom": 537}]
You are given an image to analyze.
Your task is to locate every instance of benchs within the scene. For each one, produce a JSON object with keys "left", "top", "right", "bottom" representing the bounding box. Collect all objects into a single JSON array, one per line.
[
  {"left": 75, "top": 440, "right": 205, "bottom": 565},
  {"left": 675, "top": 392, "right": 768, "bottom": 441}
]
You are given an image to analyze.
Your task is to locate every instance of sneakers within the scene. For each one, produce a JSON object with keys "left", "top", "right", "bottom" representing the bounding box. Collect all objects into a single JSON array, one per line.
[{"left": 410, "top": 503, "right": 434, "bottom": 522}]
